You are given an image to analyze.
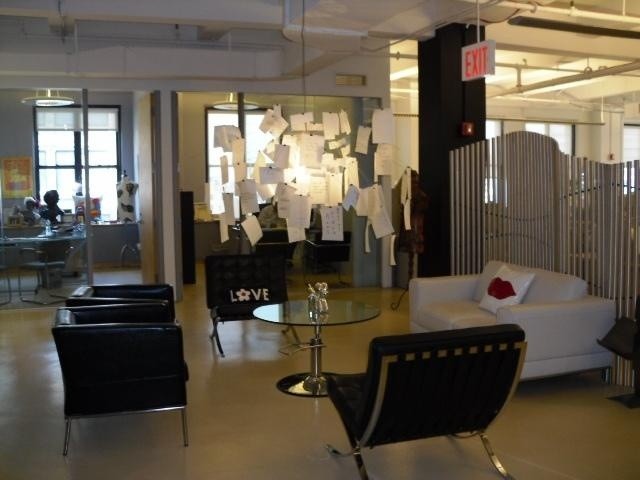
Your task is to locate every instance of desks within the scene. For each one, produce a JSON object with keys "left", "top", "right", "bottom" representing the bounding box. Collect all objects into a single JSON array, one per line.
[{"left": 0, "top": 236, "right": 89, "bottom": 289}]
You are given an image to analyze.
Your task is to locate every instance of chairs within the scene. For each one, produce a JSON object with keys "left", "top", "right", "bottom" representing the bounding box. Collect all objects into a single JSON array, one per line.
[
  {"left": 1, "top": 250, "right": 12, "bottom": 306},
  {"left": 304, "top": 231, "right": 353, "bottom": 281},
  {"left": 52, "top": 302, "right": 188, "bottom": 455},
  {"left": 322, "top": 323, "right": 528, "bottom": 479},
  {"left": 257, "top": 230, "right": 297, "bottom": 275},
  {"left": 205, "top": 252, "right": 300, "bottom": 357},
  {"left": 67, "top": 284, "right": 176, "bottom": 305},
  {"left": 253, "top": 203, "right": 273, "bottom": 217},
  {"left": 17, "top": 247, "right": 76, "bottom": 306}
]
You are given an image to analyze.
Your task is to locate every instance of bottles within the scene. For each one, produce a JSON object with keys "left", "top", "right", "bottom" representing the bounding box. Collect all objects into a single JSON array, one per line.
[
  {"left": 76, "top": 205, "right": 84, "bottom": 224},
  {"left": 46, "top": 216, "right": 52, "bottom": 235}
]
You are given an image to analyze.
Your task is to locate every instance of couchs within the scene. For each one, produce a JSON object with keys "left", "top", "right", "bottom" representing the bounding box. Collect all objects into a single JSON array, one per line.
[{"left": 409, "top": 260, "right": 615, "bottom": 383}]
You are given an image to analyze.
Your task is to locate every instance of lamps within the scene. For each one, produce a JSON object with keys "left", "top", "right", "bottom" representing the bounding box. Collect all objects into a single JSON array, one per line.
[
  {"left": 214, "top": 91, "right": 258, "bottom": 111},
  {"left": 20, "top": 89, "right": 75, "bottom": 108}
]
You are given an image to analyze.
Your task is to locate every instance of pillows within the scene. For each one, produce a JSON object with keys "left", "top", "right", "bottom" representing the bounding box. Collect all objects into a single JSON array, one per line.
[
  {"left": 519, "top": 268, "right": 588, "bottom": 304},
  {"left": 477, "top": 263, "right": 536, "bottom": 315},
  {"left": 472, "top": 259, "right": 519, "bottom": 303}
]
